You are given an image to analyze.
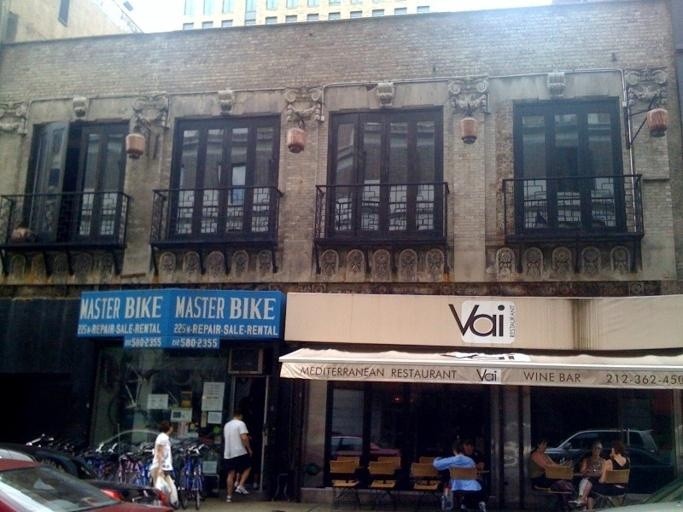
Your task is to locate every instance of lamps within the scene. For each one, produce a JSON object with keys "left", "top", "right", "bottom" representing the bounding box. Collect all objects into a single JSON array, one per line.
[
  {"left": 625, "top": 93, "right": 669, "bottom": 151},
  {"left": 125, "top": 113, "right": 151, "bottom": 159},
  {"left": 286, "top": 111, "right": 306, "bottom": 152},
  {"left": 459, "top": 105, "right": 478, "bottom": 143}
]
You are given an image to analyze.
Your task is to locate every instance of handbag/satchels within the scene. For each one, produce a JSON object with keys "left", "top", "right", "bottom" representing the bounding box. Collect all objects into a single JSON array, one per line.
[{"left": 155, "top": 471, "right": 177, "bottom": 504}]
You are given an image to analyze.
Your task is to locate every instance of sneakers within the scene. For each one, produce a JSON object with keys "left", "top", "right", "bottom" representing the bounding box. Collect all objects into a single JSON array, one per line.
[
  {"left": 234, "top": 481, "right": 250, "bottom": 494},
  {"left": 226, "top": 496, "right": 232, "bottom": 502}
]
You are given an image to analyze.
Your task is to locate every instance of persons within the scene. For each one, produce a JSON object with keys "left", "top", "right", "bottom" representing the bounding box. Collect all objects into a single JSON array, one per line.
[
  {"left": 222, "top": 408, "right": 253, "bottom": 503},
  {"left": 443, "top": 437, "right": 487, "bottom": 511},
  {"left": 575, "top": 441, "right": 607, "bottom": 511},
  {"left": 527, "top": 438, "right": 575, "bottom": 511},
  {"left": 432, "top": 440, "right": 482, "bottom": 512},
  {"left": 567, "top": 439, "right": 631, "bottom": 511},
  {"left": 148, "top": 418, "right": 179, "bottom": 510}
]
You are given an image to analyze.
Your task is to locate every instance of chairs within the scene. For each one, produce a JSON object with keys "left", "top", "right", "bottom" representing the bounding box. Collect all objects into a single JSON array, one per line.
[
  {"left": 329, "top": 455, "right": 482, "bottom": 510},
  {"left": 534, "top": 455, "right": 631, "bottom": 511}
]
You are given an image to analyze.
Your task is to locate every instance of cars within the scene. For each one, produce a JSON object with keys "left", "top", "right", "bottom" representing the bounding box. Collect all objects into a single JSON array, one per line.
[
  {"left": 0, "top": 446, "right": 175, "bottom": 512},
  {"left": 573, "top": 445, "right": 675, "bottom": 494},
  {"left": 330, "top": 435, "right": 399, "bottom": 459}
]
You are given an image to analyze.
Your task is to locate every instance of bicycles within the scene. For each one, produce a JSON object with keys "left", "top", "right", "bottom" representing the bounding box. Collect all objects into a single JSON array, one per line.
[{"left": 26, "top": 434, "right": 211, "bottom": 510}]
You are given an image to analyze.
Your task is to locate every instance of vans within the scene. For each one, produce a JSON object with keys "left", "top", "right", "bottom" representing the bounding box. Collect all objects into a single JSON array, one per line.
[{"left": 544, "top": 428, "right": 663, "bottom": 463}]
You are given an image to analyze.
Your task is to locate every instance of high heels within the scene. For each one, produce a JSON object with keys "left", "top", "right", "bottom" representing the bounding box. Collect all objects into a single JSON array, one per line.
[{"left": 567, "top": 496, "right": 587, "bottom": 509}]
